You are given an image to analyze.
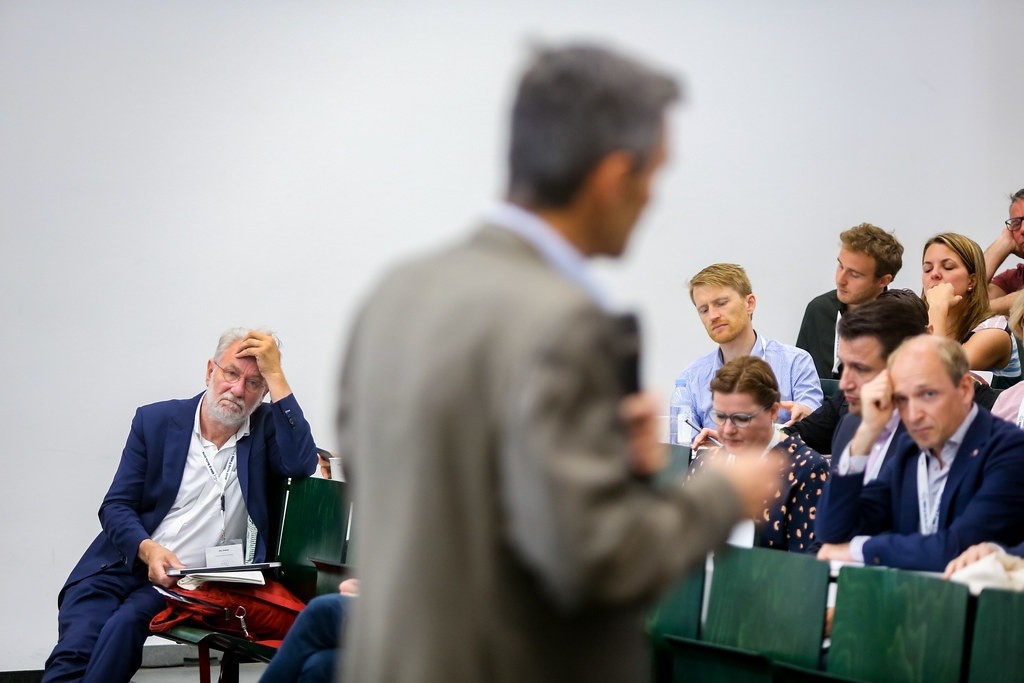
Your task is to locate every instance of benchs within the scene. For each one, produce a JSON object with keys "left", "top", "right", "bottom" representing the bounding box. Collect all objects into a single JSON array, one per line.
[
  {"left": 153, "top": 478, "right": 354, "bottom": 683},
  {"left": 644, "top": 441, "right": 1024, "bottom": 683}
]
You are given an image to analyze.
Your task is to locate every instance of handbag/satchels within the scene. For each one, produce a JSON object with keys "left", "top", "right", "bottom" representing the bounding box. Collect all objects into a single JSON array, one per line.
[{"left": 148, "top": 582, "right": 307, "bottom": 650}]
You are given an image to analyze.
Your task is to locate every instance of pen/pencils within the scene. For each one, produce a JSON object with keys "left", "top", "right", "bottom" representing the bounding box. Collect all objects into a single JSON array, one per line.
[{"left": 686, "top": 419, "right": 723, "bottom": 447}]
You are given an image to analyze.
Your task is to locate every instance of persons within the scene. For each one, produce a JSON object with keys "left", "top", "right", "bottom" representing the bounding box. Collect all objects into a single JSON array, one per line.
[
  {"left": 981, "top": 190, "right": 1024, "bottom": 315},
  {"left": 921, "top": 233, "right": 1024, "bottom": 390},
  {"left": 815, "top": 288, "right": 934, "bottom": 485},
  {"left": 39, "top": 326, "right": 318, "bottom": 683},
  {"left": 811, "top": 334, "right": 1024, "bottom": 648},
  {"left": 677, "top": 355, "right": 830, "bottom": 556},
  {"left": 796, "top": 223, "right": 905, "bottom": 381},
  {"left": 989, "top": 291, "right": 1024, "bottom": 430},
  {"left": 668, "top": 262, "right": 823, "bottom": 461},
  {"left": 254, "top": 43, "right": 789, "bottom": 683}
]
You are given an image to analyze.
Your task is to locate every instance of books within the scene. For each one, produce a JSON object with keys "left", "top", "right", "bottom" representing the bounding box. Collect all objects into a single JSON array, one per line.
[{"left": 186, "top": 570, "right": 265, "bottom": 587}]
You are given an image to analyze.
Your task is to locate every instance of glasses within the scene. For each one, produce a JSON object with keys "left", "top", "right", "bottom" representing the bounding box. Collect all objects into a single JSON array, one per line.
[
  {"left": 215, "top": 361, "right": 266, "bottom": 395},
  {"left": 1005, "top": 216, "right": 1024, "bottom": 231},
  {"left": 709, "top": 401, "right": 771, "bottom": 429}
]
